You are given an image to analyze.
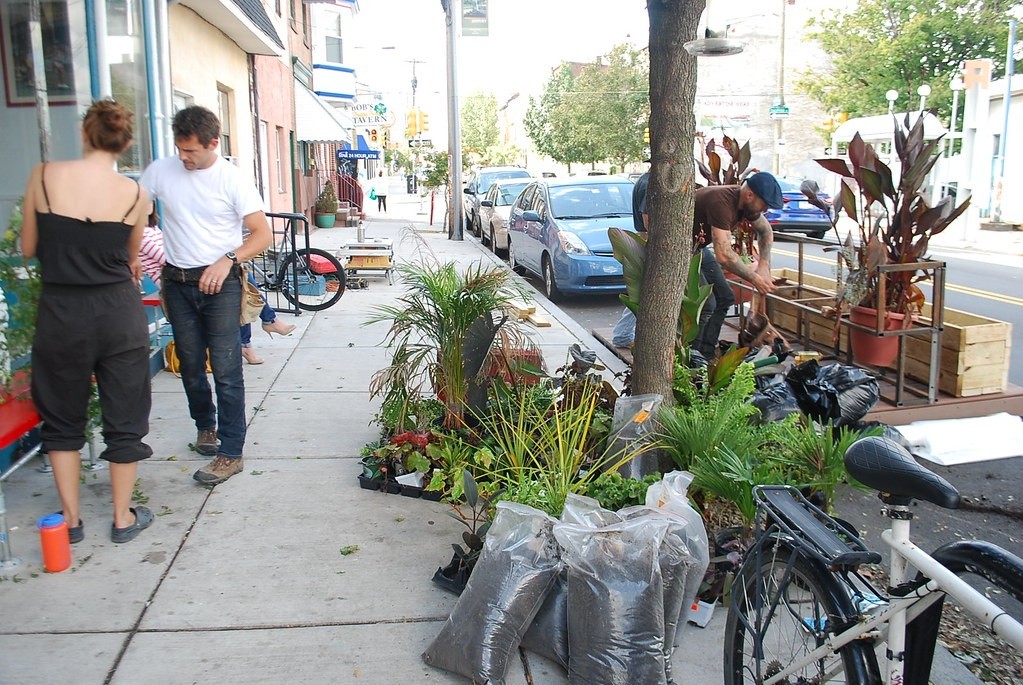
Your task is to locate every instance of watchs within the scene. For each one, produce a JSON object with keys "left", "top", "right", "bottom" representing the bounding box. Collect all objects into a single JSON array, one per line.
[{"left": 225, "top": 252, "right": 237, "bottom": 267}]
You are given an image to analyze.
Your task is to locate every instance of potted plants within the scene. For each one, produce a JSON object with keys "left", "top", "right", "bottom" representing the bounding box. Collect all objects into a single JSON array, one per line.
[
  {"left": 357, "top": 104, "right": 973, "bottom": 630},
  {"left": 313, "top": 180, "right": 338, "bottom": 227}
]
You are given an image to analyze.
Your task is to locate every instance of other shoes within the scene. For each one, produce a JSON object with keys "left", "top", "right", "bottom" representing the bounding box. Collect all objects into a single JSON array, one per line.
[{"left": 615, "top": 338, "right": 635, "bottom": 349}]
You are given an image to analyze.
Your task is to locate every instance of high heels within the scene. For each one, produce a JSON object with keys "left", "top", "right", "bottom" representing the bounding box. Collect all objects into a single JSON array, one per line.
[
  {"left": 262, "top": 319, "right": 296, "bottom": 340},
  {"left": 240, "top": 347, "right": 264, "bottom": 364}
]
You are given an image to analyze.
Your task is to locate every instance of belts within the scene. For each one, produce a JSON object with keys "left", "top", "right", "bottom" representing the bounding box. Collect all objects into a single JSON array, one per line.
[{"left": 163, "top": 266, "right": 243, "bottom": 283}]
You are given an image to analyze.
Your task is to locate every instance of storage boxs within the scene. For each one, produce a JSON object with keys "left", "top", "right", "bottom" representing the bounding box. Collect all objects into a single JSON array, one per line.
[{"left": 754, "top": 262, "right": 1013, "bottom": 399}]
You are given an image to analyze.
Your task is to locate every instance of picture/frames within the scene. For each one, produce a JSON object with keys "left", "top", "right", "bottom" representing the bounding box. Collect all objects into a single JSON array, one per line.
[{"left": 0, "top": 0, "right": 77, "bottom": 107}]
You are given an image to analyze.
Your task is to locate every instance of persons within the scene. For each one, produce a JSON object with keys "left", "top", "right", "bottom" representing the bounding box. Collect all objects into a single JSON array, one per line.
[
  {"left": 692, "top": 172, "right": 783, "bottom": 368},
  {"left": 373, "top": 173, "right": 387, "bottom": 212},
  {"left": 139, "top": 106, "right": 273, "bottom": 485},
  {"left": 22, "top": 99, "right": 154, "bottom": 543},
  {"left": 632, "top": 167, "right": 648, "bottom": 233},
  {"left": 139, "top": 202, "right": 296, "bottom": 364}
]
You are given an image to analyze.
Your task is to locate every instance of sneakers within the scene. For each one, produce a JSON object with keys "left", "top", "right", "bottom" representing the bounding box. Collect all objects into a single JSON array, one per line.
[
  {"left": 196, "top": 428, "right": 217, "bottom": 455},
  {"left": 192, "top": 455, "right": 243, "bottom": 485}
]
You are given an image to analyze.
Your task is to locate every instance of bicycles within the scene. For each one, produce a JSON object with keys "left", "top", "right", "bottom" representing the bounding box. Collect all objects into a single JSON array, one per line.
[
  {"left": 241, "top": 213, "right": 346, "bottom": 311},
  {"left": 721, "top": 435, "right": 1023, "bottom": 685}
]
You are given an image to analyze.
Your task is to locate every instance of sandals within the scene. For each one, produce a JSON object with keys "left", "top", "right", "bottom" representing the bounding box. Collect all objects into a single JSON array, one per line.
[
  {"left": 55, "top": 510, "right": 84, "bottom": 543},
  {"left": 111, "top": 505, "right": 154, "bottom": 543}
]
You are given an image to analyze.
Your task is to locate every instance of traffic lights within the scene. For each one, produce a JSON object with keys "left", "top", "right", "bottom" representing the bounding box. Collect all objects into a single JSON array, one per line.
[
  {"left": 380, "top": 131, "right": 387, "bottom": 147},
  {"left": 419, "top": 111, "right": 429, "bottom": 132},
  {"left": 406, "top": 111, "right": 416, "bottom": 136},
  {"left": 370, "top": 128, "right": 378, "bottom": 145},
  {"left": 644, "top": 128, "right": 650, "bottom": 144}
]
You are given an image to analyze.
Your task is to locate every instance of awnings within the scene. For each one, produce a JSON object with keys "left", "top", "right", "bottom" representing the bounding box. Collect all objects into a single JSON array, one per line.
[{"left": 295, "top": 81, "right": 353, "bottom": 144}]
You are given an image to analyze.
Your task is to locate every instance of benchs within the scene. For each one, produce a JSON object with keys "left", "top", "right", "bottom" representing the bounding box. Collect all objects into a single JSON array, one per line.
[
  {"left": 142, "top": 290, "right": 174, "bottom": 347},
  {"left": 0, "top": 363, "right": 102, "bottom": 561}
]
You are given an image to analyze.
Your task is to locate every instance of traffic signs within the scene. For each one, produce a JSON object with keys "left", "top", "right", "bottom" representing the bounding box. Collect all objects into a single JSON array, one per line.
[{"left": 408, "top": 139, "right": 432, "bottom": 147}]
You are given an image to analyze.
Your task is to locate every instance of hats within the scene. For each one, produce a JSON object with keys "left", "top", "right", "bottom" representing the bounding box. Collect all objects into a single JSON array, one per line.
[{"left": 747, "top": 171, "right": 783, "bottom": 210}]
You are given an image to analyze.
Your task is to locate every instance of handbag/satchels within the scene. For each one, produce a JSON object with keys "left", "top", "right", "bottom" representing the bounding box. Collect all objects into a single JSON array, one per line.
[
  {"left": 164, "top": 341, "right": 213, "bottom": 378},
  {"left": 238, "top": 266, "right": 265, "bottom": 326}
]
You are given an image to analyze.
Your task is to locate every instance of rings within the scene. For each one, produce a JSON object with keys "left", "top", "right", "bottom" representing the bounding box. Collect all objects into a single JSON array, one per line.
[{"left": 211, "top": 280, "right": 216, "bottom": 283}]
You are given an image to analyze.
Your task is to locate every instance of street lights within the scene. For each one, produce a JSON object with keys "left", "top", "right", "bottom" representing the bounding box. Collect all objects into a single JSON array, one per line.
[{"left": 943, "top": 79, "right": 963, "bottom": 199}]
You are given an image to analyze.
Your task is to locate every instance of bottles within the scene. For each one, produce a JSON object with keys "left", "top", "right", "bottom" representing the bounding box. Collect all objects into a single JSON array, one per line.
[
  {"left": 37, "top": 514, "right": 70, "bottom": 572},
  {"left": 357, "top": 221, "right": 365, "bottom": 243},
  {"left": 797, "top": 351, "right": 819, "bottom": 362}
]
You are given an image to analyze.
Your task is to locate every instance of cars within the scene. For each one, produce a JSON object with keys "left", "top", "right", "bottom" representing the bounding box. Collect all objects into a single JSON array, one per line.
[
  {"left": 760, "top": 177, "right": 835, "bottom": 239},
  {"left": 507, "top": 175, "right": 637, "bottom": 304},
  {"left": 461, "top": 164, "right": 643, "bottom": 257}
]
